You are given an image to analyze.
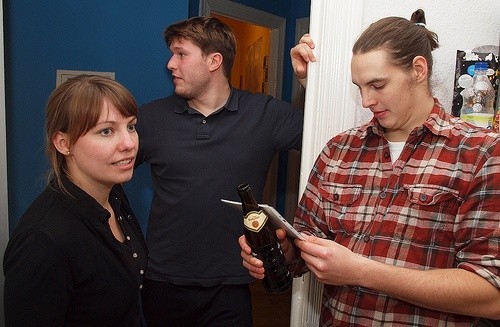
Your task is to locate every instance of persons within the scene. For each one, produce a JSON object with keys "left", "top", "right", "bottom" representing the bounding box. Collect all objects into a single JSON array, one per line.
[
  {"left": 3, "top": 73, "right": 148, "bottom": 327},
  {"left": 133, "top": 16, "right": 317, "bottom": 326},
  {"left": 239, "top": 9, "right": 500, "bottom": 327}
]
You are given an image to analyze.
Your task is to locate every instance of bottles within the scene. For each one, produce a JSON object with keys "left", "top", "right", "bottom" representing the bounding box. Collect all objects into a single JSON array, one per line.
[
  {"left": 460, "top": 62, "right": 495, "bottom": 130},
  {"left": 236, "top": 183, "right": 292, "bottom": 294}
]
